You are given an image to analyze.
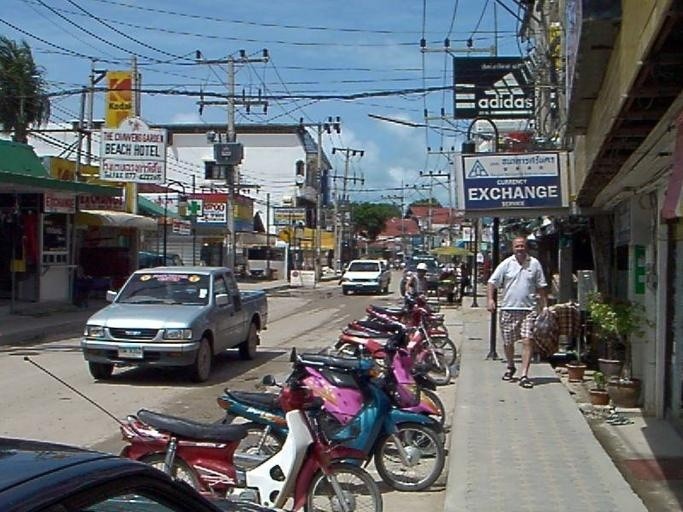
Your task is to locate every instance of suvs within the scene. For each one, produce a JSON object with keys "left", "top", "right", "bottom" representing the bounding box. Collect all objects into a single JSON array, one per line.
[
  {"left": 0, "top": 356, "right": 225, "bottom": 512},
  {"left": 139, "top": 251, "right": 183, "bottom": 269},
  {"left": 401, "top": 256, "right": 444, "bottom": 295}
]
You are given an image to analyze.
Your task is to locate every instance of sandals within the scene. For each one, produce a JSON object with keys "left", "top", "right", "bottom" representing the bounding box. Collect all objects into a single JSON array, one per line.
[
  {"left": 502, "top": 365, "right": 517, "bottom": 381},
  {"left": 520, "top": 376, "right": 533, "bottom": 388}
]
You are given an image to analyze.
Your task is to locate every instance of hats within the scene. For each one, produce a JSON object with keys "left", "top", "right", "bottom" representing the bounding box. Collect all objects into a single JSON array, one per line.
[{"left": 416, "top": 262, "right": 429, "bottom": 272}]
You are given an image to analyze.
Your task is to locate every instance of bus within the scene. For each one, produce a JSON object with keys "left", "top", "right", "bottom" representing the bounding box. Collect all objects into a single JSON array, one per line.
[{"left": 242, "top": 244, "right": 285, "bottom": 279}]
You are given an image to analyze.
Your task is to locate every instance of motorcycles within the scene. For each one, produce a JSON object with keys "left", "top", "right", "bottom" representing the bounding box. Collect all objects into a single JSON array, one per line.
[
  {"left": 112, "top": 347, "right": 382, "bottom": 511},
  {"left": 217, "top": 345, "right": 445, "bottom": 492},
  {"left": 286, "top": 289, "right": 457, "bottom": 451}
]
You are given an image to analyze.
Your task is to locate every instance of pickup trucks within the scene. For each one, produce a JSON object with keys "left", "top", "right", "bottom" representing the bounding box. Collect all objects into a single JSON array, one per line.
[{"left": 80, "top": 266, "right": 268, "bottom": 382}]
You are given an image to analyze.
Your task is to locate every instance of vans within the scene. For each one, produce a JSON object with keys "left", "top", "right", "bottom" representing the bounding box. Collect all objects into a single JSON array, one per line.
[{"left": 342, "top": 260, "right": 390, "bottom": 294}]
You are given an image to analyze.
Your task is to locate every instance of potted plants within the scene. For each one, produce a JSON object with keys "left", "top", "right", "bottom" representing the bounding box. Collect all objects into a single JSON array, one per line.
[
  {"left": 597, "top": 338, "right": 624, "bottom": 379},
  {"left": 585, "top": 290, "right": 655, "bottom": 407},
  {"left": 566, "top": 358, "right": 587, "bottom": 382},
  {"left": 589, "top": 370, "right": 610, "bottom": 406}
]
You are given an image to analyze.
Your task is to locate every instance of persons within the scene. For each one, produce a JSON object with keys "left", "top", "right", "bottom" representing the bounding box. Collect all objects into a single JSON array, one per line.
[
  {"left": 338, "top": 263, "right": 347, "bottom": 285},
  {"left": 461, "top": 266, "right": 470, "bottom": 296},
  {"left": 488, "top": 235, "right": 550, "bottom": 388},
  {"left": 405, "top": 262, "right": 429, "bottom": 296},
  {"left": 482, "top": 256, "right": 491, "bottom": 283}
]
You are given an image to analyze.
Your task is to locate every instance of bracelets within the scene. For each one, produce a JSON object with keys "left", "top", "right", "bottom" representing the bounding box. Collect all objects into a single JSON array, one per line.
[{"left": 543, "top": 306, "right": 548, "bottom": 310}]
